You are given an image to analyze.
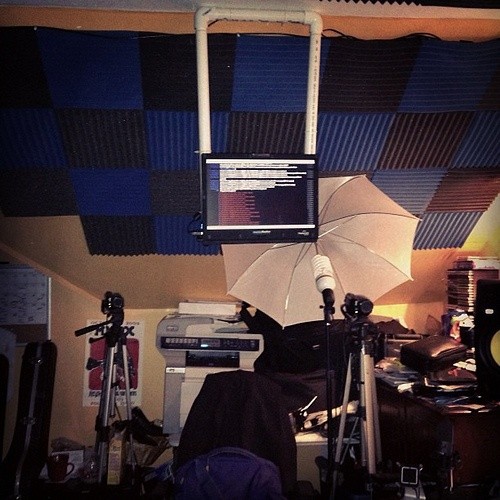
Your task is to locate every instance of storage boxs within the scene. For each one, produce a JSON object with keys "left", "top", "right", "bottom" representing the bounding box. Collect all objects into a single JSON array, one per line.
[{"left": 46, "top": 446, "right": 85, "bottom": 483}]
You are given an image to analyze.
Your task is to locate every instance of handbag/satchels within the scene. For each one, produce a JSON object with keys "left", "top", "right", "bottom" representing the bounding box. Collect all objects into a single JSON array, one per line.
[{"left": 400, "top": 334, "right": 468, "bottom": 375}]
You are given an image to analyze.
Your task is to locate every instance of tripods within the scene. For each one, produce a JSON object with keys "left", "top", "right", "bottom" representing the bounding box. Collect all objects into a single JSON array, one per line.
[
  {"left": 330, "top": 318, "right": 382, "bottom": 500},
  {"left": 74, "top": 311, "right": 136, "bottom": 487}
]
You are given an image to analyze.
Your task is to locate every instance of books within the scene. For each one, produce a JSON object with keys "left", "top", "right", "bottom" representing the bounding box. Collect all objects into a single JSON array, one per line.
[{"left": 453, "top": 255, "right": 500, "bottom": 271}]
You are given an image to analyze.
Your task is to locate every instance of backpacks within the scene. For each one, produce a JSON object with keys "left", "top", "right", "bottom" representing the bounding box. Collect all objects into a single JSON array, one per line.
[{"left": 167, "top": 448, "right": 283, "bottom": 500}]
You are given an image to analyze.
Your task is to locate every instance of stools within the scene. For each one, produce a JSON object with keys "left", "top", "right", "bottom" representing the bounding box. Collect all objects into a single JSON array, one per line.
[{"left": 289, "top": 406, "right": 359, "bottom": 496}]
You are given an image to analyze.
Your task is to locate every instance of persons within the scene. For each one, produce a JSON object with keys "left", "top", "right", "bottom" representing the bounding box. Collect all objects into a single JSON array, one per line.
[
  {"left": 169, "top": 428, "right": 286, "bottom": 500},
  {"left": 239, "top": 301, "right": 323, "bottom": 415}
]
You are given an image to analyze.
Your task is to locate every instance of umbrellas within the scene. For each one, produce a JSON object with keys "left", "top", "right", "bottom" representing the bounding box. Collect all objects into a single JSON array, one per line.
[{"left": 220, "top": 173, "right": 422, "bottom": 330}]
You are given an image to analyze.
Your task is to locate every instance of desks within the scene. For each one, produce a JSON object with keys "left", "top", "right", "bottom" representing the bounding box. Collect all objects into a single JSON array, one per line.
[{"left": 373, "top": 355, "right": 500, "bottom": 500}]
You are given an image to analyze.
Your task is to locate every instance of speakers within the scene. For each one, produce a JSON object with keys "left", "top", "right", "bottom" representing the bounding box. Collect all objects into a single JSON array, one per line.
[{"left": 473, "top": 279, "right": 500, "bottom": 401}]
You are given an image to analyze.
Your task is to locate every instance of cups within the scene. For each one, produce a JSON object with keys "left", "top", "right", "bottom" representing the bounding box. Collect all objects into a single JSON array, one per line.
[{"left": 47, "top": 454, "right": 74, "bottom": 480}]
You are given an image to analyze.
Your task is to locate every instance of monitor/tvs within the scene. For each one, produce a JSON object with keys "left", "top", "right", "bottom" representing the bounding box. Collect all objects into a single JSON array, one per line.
[{"left": 200, "top": 153, "right": 319, "bottom": 243}]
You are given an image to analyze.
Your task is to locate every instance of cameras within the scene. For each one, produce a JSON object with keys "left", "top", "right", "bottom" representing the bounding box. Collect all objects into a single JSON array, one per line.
[
  {"left": 344, "top": 294, "right": 373, "bottom": 321},
  {"left": 101, "top": 291, "right": 124, "bottom": 314}
]
